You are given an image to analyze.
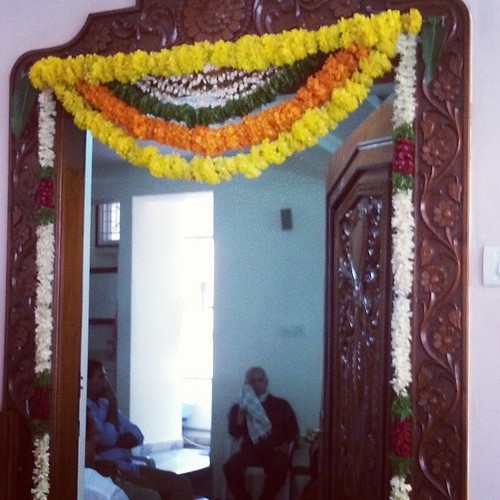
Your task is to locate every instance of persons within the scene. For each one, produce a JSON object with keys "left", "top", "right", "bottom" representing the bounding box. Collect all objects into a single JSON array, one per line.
[
  {"left": 86, "top": 406, "right": 135, "bottom": 500},
  {"left": 224, "top": 366, "right": 302, "bottom": 500},
  {"left": 88, "top": 359, "right": 196, "bottom": 500}
]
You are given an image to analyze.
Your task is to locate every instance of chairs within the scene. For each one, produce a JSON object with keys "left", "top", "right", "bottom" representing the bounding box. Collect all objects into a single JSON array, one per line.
[{"left": 221, "top": 435, "right": 291, "bottom": 500}]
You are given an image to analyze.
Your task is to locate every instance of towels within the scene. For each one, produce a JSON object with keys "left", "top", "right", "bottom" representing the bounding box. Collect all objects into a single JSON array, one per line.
[{"left": 240, "top": 382, "right": 272, "bottom": 444}]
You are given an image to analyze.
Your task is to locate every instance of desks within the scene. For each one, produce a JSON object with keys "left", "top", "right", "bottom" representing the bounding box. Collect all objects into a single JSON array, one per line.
[{"left": 132, "top": 446, "right": 211, "bottom": 500}]
